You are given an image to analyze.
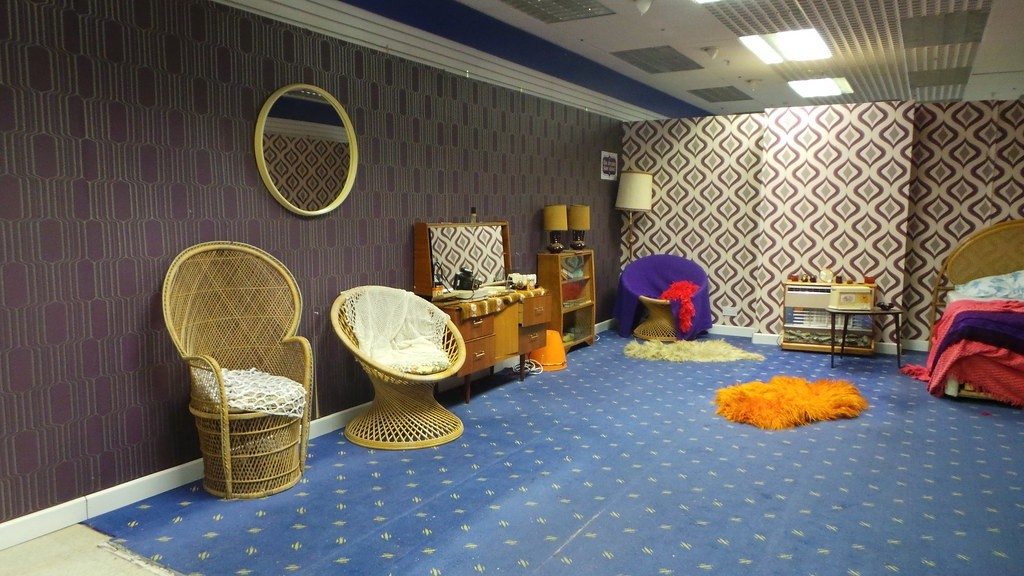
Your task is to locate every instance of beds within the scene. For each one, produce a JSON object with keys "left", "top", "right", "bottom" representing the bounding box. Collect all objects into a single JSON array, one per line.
[{"left": 901, "top": 220, "right": 1024, "bottom": 405}]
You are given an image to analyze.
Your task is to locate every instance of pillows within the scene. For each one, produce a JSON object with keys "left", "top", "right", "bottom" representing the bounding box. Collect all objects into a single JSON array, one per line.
[{"left": 954, "top": 269, "right": 1024, "bottom": 299}]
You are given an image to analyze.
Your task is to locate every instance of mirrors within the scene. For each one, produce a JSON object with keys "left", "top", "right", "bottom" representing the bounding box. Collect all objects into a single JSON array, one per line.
[{"left": 255, "top": 84, "right": 359, "bottom": 216}]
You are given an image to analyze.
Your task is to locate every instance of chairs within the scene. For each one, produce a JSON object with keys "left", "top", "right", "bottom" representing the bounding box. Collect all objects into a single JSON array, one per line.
[
  {"left": 613, "top": 253, "right": 713, "bottom": 340},
  {"left": 160, "top": 240, "right": 306, "bottom": 497},
  {"left": 332, "top": 284, "right": 465, "bottom": 450}
]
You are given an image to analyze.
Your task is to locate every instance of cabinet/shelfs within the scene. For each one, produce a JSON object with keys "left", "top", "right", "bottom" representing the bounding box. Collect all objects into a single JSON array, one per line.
[
  {"left": 413, "top": 222, "right": 552, "bottom": 404},
  {"left": 538, "top": 250, "right": 595, "bottom": 354},
  {"left": 782, "top": 280, "right": 877, "bottom": 358}
]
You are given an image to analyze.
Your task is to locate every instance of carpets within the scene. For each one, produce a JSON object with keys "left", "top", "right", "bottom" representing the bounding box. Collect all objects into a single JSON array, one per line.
[
  {"left": 623, "top": 336, "right": 766, "bottom": 363},
  {"left": 715, "top": 375, "right": 868, "bottom": 430}
]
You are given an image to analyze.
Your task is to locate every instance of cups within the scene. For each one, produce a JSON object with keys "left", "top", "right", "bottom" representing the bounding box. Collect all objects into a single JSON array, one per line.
[{"left": 864, "top": 276, "right": 876, "bottom": 284}]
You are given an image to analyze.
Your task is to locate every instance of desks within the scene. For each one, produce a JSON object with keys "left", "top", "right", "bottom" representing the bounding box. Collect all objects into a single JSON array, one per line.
[{"left": 825, "top": 307, "right": 904, "bottom": 369}]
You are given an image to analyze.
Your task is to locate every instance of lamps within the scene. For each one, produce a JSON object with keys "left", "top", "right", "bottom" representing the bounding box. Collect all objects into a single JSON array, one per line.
[
  {"left": 635, "top": 0, "right": 652, "bottom": 17},
  {"left": 544, "top": 204, "right": 567, "bottom": 254},
  {"left": 700, "top": 46, "right": 720, "bottom": 59},
  {"left": 615, "top": 172, "right": 656, "bottom": 263},
  {"left": 570, "top": 204, "right": 591, "bottom": 250}
]
